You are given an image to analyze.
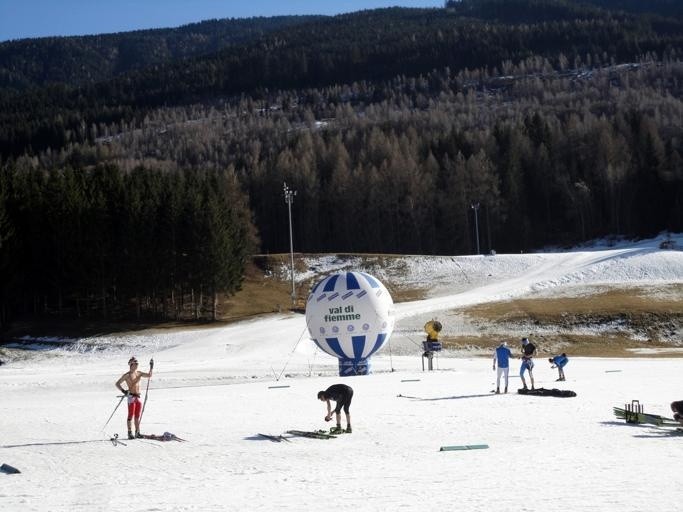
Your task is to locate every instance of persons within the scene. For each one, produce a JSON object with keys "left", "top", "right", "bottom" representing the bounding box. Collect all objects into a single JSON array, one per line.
[
  {"left": 520, "top": 337, "right": 538, "bottom": 390},
  {"left": 493, "top": 339, "right": 520, "bottom": 394},
  {"left": 549, "top": 353, "right": 569, "bottom": 381},
  {"left": 318, "top": 384, "right": 353, "bottom": 434},
  {"left": 115, "top": 356, "right": 154, "bottom": 439}
]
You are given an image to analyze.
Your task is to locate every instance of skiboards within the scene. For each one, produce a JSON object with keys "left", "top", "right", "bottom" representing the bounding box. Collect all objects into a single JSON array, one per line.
[{"left": 285, "top": 429, "right": 336, "bottom": 439}]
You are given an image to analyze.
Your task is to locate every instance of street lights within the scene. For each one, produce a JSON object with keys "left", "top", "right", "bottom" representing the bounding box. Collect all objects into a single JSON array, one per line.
[
  {"left": 470, "top": 202, "right": 481, "bottom": 254},
  {"left": 282, "top": 181, "right": 300, "bottom": 306}
]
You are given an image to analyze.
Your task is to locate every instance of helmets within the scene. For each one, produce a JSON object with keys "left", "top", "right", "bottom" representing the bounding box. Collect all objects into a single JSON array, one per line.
[{"left": 128, "top": 356, "right": 139, "bottom": 366}]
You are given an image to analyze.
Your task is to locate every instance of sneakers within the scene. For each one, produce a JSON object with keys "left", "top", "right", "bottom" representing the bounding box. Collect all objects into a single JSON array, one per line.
[
  {"left": 556, "top": 378, "right": 566, "bottom": 382},
  {"left": 329, "top": 427, "right": 353, "bottom": 435},
  {"left": 128, "top": 431, "right": 144, "bottom": 439}
]
